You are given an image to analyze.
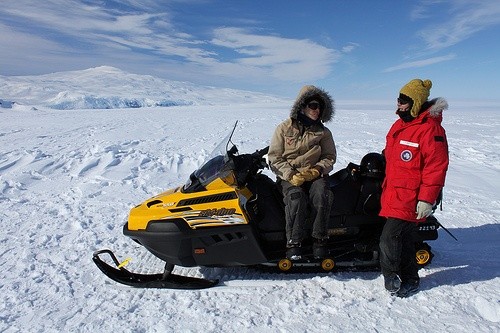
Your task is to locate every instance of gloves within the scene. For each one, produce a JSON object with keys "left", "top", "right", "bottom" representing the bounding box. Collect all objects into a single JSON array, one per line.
[
  {"left": 298, "top": 168, "right": 319, "bottom": 181},
  {"left": 415, "top": 201, "right": 432, "bottom": 220},
  {"left": 288, "top": 171, "right": 305, "bottom": 187}
]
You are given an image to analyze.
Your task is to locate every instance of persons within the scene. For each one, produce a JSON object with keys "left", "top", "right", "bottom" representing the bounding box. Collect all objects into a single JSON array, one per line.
[
  {"left": 268, "top": 84, "right": 337, "bottom": 260},
  {"left": 378, "top": 79, "right": 449, "bottom": 297}
]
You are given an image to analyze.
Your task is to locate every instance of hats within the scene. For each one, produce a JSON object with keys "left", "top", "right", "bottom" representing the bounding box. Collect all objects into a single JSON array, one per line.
[{"left": 400, "top": 78, "right": 432, "bottom": 117}]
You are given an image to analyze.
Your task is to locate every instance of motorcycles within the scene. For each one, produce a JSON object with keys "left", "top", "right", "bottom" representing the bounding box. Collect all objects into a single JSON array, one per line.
[{"left": 91, "top": 120, "right": 459, "bottom": 290}]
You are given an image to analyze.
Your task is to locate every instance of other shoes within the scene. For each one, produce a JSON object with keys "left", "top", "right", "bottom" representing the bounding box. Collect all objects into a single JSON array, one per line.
[
  {"left": 398, "top": 278, "right": 419, "bottom": 297},
  {"left": 383, "top": 273, "right": 402, "bottom": 293},
  {"left": 287, "top": 247, "right": 302, "bottom": 260},
  {"left": 312, "top": 241, "right": 330, "bottom": 257}
]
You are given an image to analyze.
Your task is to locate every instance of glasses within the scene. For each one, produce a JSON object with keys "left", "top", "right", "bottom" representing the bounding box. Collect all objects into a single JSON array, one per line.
[
  {"left": 307, "top": 103, "right": 322, "bottom": 110},
  {"left": 397, "top": 98, "right": 408, "bottom": 105}
]
